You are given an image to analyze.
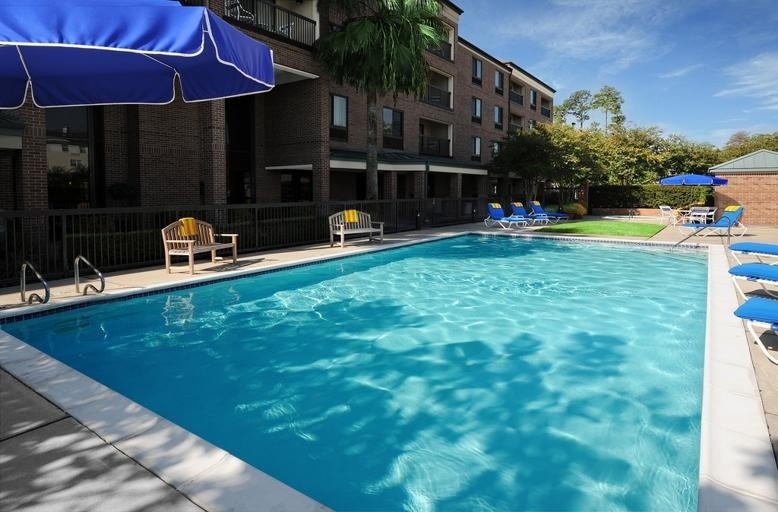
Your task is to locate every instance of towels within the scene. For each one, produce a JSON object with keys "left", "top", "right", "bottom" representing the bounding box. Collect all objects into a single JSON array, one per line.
[
  {"left": 177, "top": 218, "right": 198, "bottom": 237},
  {"left": 344, "top": 209, "right": 359, "bottom": 223}
]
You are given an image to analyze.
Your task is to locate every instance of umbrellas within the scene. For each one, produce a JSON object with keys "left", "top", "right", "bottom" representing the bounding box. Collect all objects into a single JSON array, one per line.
[
  {"left": 660, "top": 173, "right": 728, "bottom": 210},
  {"left": 0, "top": 1, "right": 276, "bottom": 111}
]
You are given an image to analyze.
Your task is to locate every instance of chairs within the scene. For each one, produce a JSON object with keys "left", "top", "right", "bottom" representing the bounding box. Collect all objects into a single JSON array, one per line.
[
  {"left": 728, "top": 241, "right": 778, "bottom": 356},
  {"left": 678, "top": 205, "right": 747, "bottom": 241},
  {"left": 225, "top": 1, "right": 294, "bottom": 39},
  {"left": 658, "top": 205, "right": 717, "bottom": 226},
  {"left": 483, "top": 200, "right": 571, "bottom": 230}
]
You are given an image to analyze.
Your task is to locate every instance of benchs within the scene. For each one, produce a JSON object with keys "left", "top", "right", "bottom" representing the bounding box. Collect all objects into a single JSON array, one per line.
[
  {"left": 159, "top": 217, "right": 240, "bottom": 274},
  {"left": 329, "top": 209, "right": 384, "bottom": 248}
]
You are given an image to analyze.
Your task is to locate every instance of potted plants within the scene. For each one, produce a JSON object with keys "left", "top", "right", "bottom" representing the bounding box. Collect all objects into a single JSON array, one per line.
[{"left": 561, "top": 204, "right": 586, "bottom": 219}]
